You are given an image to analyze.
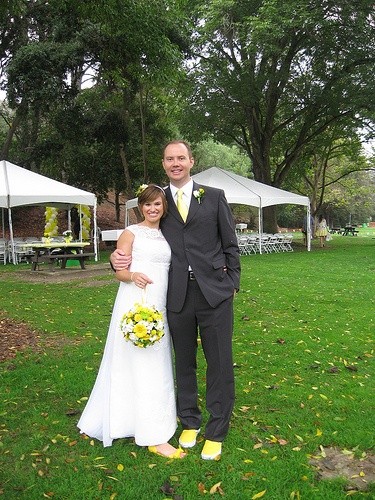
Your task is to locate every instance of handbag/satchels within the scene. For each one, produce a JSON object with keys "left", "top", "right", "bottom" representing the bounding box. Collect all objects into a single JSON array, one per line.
[{"left": 326, "top": 232, "right": 331, "bottom": 242}]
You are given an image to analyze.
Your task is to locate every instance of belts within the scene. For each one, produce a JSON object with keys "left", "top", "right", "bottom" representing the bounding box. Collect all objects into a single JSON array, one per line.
[{"left": 188, "top": 271, "right": 196, "bottom": 280}]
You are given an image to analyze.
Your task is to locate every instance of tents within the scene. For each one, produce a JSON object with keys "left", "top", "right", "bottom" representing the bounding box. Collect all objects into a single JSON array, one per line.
[
  {"left": 0, "top": 160, "right": 98, "bottom": 265},
  {"left": 125, "top": 167, "right": 311, "bottom": 254}
]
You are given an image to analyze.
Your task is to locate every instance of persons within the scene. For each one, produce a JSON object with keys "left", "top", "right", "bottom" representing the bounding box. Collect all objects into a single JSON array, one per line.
[
  {"left": 301, "top": 212, "right": 313, "bottom": 246},
  {"left": 77, "top": 183, "right": 187, "bottom": 458},
  {"left": 109, "top": 141, "right": 241, "bottom": 460},
  {"left": 316, "top": 214, "right": 329, "bottom": 248}
]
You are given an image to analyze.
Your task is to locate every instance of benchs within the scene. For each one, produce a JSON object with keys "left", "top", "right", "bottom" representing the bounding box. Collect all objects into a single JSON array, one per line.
[
  {"left": 341, "top": 230, "right": 359, "bottom": 236},
  {"left": 18, "top": 252, "right": 95, "bottom": 271}
]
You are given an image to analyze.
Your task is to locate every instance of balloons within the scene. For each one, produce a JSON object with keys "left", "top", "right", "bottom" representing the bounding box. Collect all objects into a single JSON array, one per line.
[
  {"left": 74, "top": 204, "right": 92, "bottom": 240},
  {"left": 44, "top": 207, "right": 59, "bottom": 237}
]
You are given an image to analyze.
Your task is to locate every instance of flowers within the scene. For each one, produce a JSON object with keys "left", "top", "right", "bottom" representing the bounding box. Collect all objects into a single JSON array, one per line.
[
  {"left": 120, "top": 303, "right": 166, "bottom": 348},
  {"left": 193, "top": 188, "right": 204, "bottom": 205},
  {"left": 134, "top": 183, "right": 149, "bottom": 197}
]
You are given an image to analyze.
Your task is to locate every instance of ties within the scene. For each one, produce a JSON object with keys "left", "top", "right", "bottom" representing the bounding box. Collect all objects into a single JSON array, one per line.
[{"left": 177, "top": 189, "right": 189, "bottom": 222}]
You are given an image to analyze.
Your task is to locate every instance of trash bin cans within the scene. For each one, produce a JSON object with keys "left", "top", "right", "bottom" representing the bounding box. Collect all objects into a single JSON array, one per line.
[{"left": 362, "top": 222, "right": 367, "bottom": 228}]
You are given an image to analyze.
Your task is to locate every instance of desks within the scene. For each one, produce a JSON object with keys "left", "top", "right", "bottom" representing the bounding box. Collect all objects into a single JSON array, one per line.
[
  {"left": 16, "top": 241, "right": 90, "bottom": 272},
  {"left": 343, "top": 226, "right": 355, "bottom": 235},
  {"left": 332, "top": 228, "right": 340, "bottom": 234}
]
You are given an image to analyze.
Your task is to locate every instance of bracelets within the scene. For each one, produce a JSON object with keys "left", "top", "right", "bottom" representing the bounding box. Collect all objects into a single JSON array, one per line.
[{"left": 131, "top": 272, "right": 136, "bottom": 282}]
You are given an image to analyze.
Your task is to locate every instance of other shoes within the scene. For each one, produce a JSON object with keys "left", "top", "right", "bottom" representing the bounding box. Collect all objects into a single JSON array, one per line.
[
  {"left": 179, "top": 428, "right": 200, "bottom": 447},
  {"left": 201, "top": 439, "right": 222, "bottom": 460},
  {"left": 321, "top": 243, "right": 325, "bottom": 248}
]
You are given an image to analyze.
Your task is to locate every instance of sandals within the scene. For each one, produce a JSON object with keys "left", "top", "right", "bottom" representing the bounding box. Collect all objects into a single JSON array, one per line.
[{"left": 148, "top": 442, "right": 185, "bottom": 459}]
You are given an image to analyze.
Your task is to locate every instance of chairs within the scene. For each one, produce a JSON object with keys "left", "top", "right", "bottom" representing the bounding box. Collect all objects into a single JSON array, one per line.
[
  {"left": 236, "top": 233, "right": 294, "bottom": 257},
  {"left": 0, "top": 237, "right": 38, "bottom": 265}
]
